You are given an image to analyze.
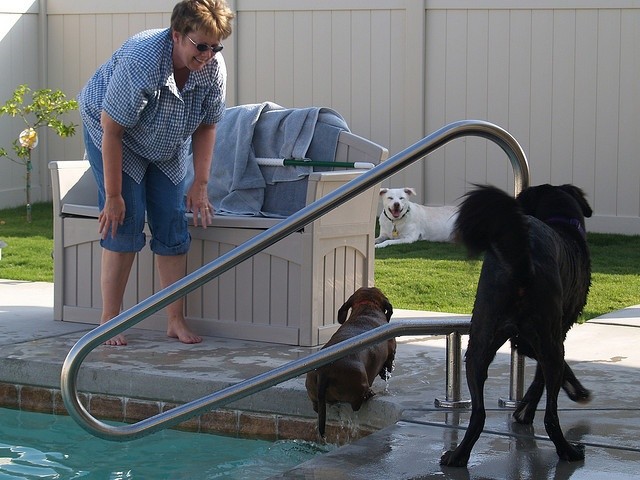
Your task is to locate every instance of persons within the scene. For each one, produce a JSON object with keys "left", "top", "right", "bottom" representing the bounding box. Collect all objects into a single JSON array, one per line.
[{"left": 78, "top": 0, "right": 237, "bottom": 346}]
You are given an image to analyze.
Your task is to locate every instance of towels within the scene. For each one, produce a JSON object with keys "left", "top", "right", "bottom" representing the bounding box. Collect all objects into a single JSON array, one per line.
[
  {"left": 203, "top": 100, "right": 283, "bottom": 216},
  {"left": 261, "top": 121, "right": 342, "bottom": 219},
  {"left": 251, "top": 107, "right": 349, "bottom": 184}
]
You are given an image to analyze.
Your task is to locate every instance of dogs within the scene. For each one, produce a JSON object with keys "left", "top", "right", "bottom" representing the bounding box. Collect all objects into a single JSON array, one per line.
[
  {"left": 438, "top": 182, "right": 598, "bottom": 469},
  {"left": 304, "top": 286, "right": 397, "bottom": 440},
  {"left": 374, "top": 186, "right": 461, "bottom": 248}
]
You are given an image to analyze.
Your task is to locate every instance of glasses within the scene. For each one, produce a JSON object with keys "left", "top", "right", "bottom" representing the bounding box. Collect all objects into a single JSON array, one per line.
[{"left": 184, "top": 32, "right": 223, "bottom": 53}]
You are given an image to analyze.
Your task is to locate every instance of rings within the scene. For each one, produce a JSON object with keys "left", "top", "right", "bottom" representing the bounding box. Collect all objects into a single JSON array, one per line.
[{"left": 205, "top": 207, "right": 209, "bottom": 210}]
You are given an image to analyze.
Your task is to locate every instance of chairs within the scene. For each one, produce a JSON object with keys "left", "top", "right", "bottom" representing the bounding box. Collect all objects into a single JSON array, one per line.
[{"left": 48, "top": 105, "right": 390, "bottom": 348}]
